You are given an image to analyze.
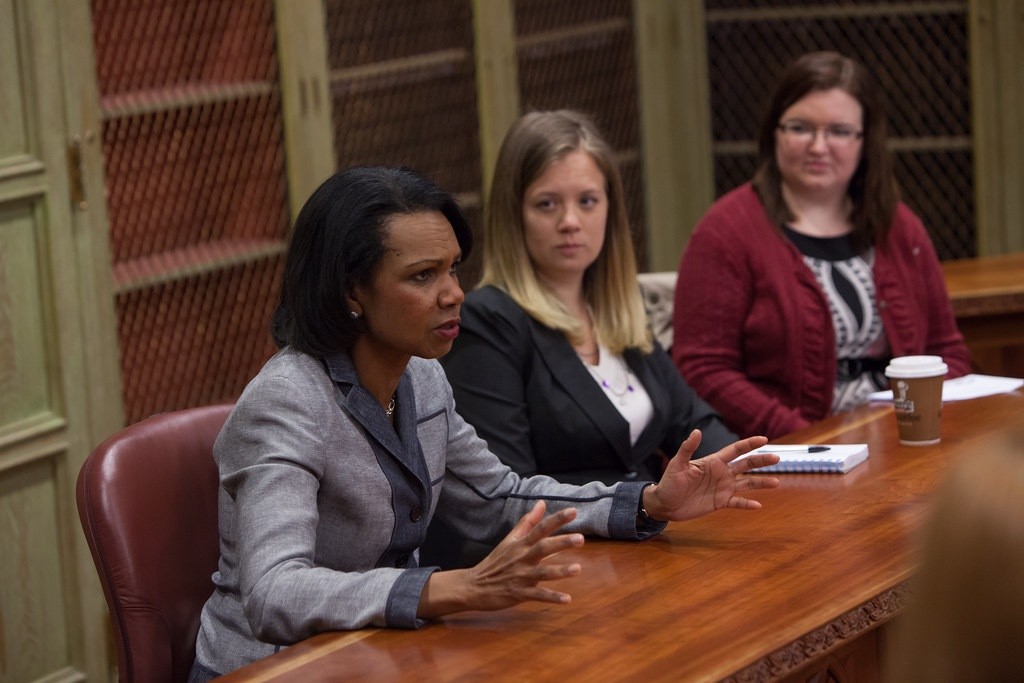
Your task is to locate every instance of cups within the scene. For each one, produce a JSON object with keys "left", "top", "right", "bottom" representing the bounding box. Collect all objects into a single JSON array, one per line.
[{"left": 884, "top": 355, "right": 948, "bottom": 444}]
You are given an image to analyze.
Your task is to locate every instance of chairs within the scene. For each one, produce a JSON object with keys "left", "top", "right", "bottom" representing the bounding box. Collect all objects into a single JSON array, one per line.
[{"left": 74, "top": 401, "right": 236, "bottom": 683}]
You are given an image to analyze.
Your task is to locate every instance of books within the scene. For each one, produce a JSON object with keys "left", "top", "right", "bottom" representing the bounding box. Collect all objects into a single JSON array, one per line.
[{"left": 727, "top": 444, "right": 869, "bottom": 474}]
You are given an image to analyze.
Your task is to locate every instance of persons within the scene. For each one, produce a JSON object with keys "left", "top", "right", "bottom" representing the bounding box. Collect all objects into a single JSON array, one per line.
[
  {"left": 191, "top": 166, "right": 780, "bottom": 679},
  {"left": 671, "top": 50, "right": 973, "bottom": 440},
  {"left": 438, "top": 110, "right": 741, "bottom": 486}
]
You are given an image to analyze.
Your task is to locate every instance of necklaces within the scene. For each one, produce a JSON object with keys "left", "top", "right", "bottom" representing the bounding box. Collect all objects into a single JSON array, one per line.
[
  {"left": 386, "top": 400, "right": 395, "bottom": 418},
  {"left": 578, "top": 350, "right": 599, "bottom": 357}
]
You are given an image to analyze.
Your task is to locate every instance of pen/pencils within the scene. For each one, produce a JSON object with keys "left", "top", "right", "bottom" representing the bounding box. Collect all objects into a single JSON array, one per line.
[{"left": 758, "top": 447, "right": 831, "bottom": 453}]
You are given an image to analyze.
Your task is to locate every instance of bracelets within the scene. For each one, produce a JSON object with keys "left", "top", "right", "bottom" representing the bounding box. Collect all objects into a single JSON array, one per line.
[{"left": 640, "top": 502, "right": 648, "bottom": 522}]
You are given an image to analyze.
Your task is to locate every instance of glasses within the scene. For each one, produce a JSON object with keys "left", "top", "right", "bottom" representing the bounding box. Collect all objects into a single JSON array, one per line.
[{"left": 778, "top": 119, "right": 864, "bottom": 141}]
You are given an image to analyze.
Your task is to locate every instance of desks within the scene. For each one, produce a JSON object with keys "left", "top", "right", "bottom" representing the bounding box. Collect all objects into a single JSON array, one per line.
[{"left": 207, "top": 377, "right": 1024, "bottom": 683}]
